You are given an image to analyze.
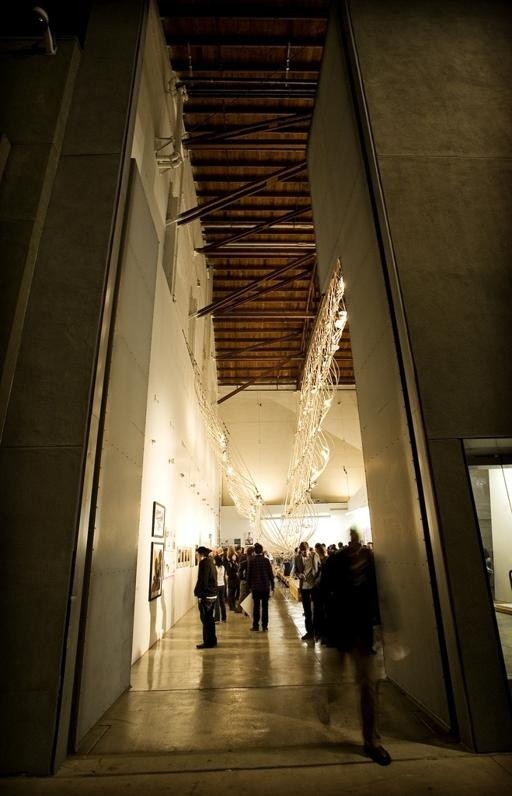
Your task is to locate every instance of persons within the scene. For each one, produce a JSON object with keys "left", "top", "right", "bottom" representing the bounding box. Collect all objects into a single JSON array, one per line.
[
  {"left": 194, "top": 543, "right": 349, "bottom": 650},
  {"left": 366, "top": 542, "right": 373, "bottom": 552},
  {"left": 199, "top": 631, "right": 273, "bottom": 703},
  {"left": 152, "top": 550, "right": 163, "bottom": 592},
  {"left": 320, "top": 526, "right": 392, "bottom": 768}
]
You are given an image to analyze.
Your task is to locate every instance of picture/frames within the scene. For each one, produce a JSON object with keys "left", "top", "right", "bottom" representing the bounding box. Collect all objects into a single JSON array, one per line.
[
  {"left": 149, "top": 542, "right": 165, "bottom": 601},
  {"left": 152, "top": 501, "right": 166, "bottom": 538}
]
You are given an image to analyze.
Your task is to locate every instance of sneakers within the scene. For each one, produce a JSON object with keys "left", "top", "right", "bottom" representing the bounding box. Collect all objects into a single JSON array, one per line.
[
  {"left": 302, "top": 632, "right": 314, "bottom": 640},
  {"left": 363, "top": 742, "right": 391, "bottom": 765},
  {"left": 215, "top": 620, "right": 226, "bottom": 624},
  {"left": 250, "top": 627, "right": 268, "bottom": 632},
  {"left": 229, "top": 607, "right": 242, "bottom": 613},
  {"left": 196, "top": 641, "right": 217, "bottom": 648}
]
